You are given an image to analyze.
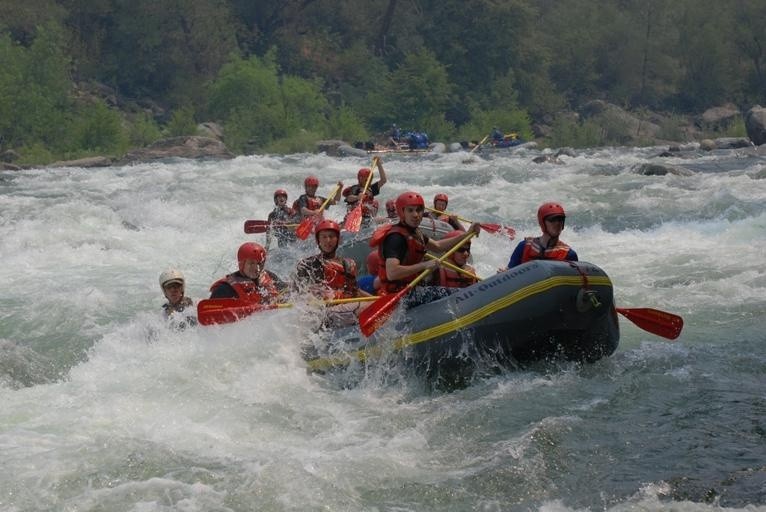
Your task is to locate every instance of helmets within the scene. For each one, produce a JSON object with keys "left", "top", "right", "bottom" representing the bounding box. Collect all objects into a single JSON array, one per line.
[
  {"left": 303, "top": 176, "right": 320, "bottom": 187},
  {"left": 357, "top": 168, "right": 374, "bottom": 178},
  {"left": 445, "top": 229, "right": 472, "bottom": 245},
  {"left": 237, "top": 242, "right": 268, "bottom": 275},
  {"left": 313, "top": 219, "right": 341, "bottom": 245},
  {"left": 396, "top": 191, "right": 426, "bottom": 223},
  {"left": 386, "top": 198, "right": 397, "bottom": 210},
  {"left": 433, "top": 192, "right": 450, "bottom": 208},
  {"left": 538, "top": 202, "right": 565, "bottom": 233},
  {"left": 273, "top": 189, "right": 288, "bottom": 204}
]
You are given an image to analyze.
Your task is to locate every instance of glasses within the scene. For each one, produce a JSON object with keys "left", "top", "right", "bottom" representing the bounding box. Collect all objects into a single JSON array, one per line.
[
  {"left": 544, "top": 216, "right": 564, "bottom": 222},
  {"left": 458, "top": 246, "right": 470, "bottom": 252}
]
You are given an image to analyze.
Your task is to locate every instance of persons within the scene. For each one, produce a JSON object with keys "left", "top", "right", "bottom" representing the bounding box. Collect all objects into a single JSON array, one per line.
[
  {"left": 155, "top": 268, "right": 199, "bottom": 333},
  {"left": 386, "top": 124, "right": 430, "bottom": 149},
  {"left": 495, "top": 202, "right": 578, "bottom": 275},
  {"left": 208, "top": 242, "right": 294, "bottom": 305},
  {"left": 263, "top": 155, "right": 480, "bottom": 337},
  {"left": 487, "top": 126, "right": 519, "bottom": 143}
]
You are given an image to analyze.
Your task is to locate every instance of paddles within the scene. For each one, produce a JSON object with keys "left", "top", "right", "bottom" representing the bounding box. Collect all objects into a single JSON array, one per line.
[
  {"left": 244, "top": 220, "right": 302, "bottom": 234},
  {"left": 359, "top": 231, "right": 477, "bottom": 336},
  {"left": 345, "top": 160, "right": 378, "bottom": 234},
  {"left": 424, "top": 208, "right": 516, "bottom": 243},
  {"left": 617, "top": 306, "right": 684, "bottom": 341},
  {"left": 198, "top": 296, "right": 384, "bottom": 326},
  {"left": 297, "top": 185, "right": 341, "bottom": 241}
]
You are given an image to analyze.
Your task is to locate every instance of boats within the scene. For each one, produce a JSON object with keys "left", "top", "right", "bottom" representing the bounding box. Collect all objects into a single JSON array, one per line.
[
  {"left": 297, "top": 259, "right": 620, "bottom": 402},
  {"left": 450, "top": 139, "right": 537, "bottom": 159},
  {"left": 258, "top": 215, "right": 455, "bottom": 279},
  {"left": 337, "top": 142, "right": 444, "bottom": 162}
]
